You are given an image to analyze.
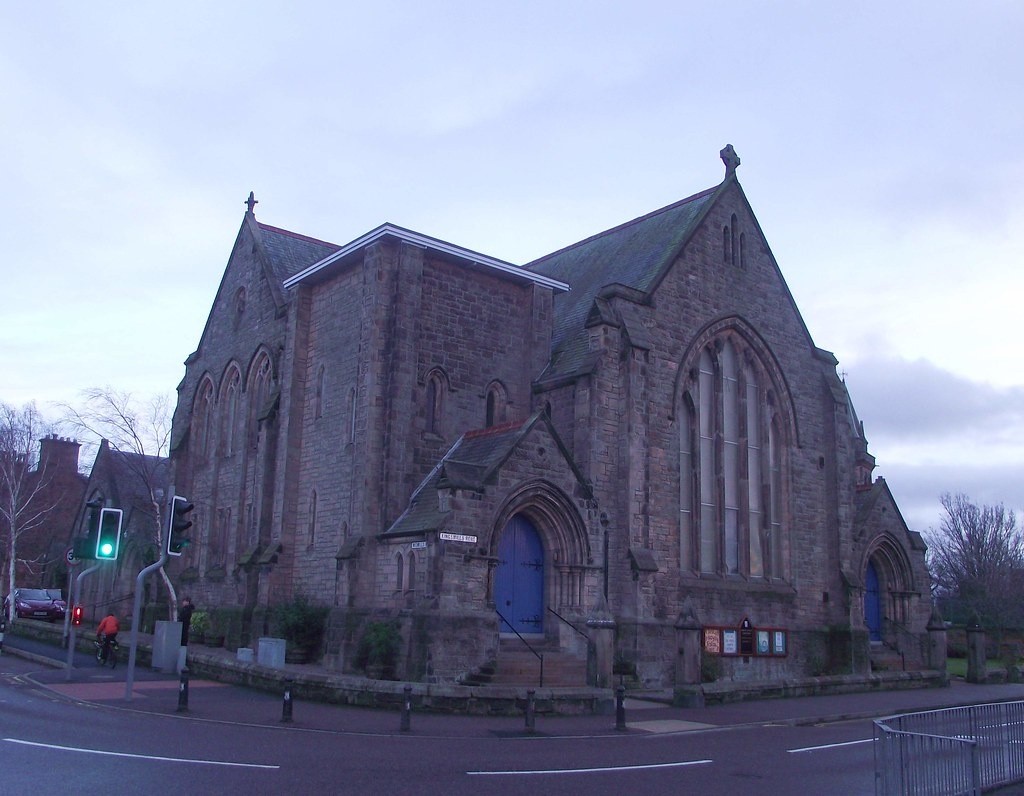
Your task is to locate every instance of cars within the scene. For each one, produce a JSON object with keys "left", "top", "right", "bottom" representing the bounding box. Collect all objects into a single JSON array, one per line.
[{"left": 5, "top": 587, "right": 57, "bottom": 623}]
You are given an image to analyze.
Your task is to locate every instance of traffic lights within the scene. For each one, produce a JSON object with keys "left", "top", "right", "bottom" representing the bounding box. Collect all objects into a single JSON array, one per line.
[
  {"left": 71, "top": 604, "right": 83, "bottom": 628},
  {"left": 164, "top": 495, "right": 194, "bottom": 558},
  {"left": 94, "top": 507, "right": 125, "bottom": 562}
]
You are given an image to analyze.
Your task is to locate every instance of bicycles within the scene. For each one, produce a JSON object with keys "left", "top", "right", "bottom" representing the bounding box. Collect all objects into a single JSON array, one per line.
[{"left": 93, "top": 631, "right": 120, "bottom": 670}]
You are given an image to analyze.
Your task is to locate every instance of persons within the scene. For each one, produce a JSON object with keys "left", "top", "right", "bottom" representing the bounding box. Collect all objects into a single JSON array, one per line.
[
  {"left": 178, "top": 597, "right": 196, "bottom": 666},
  {"left": 95, "top": 613, "right": 120, "bottom": 662}
]
